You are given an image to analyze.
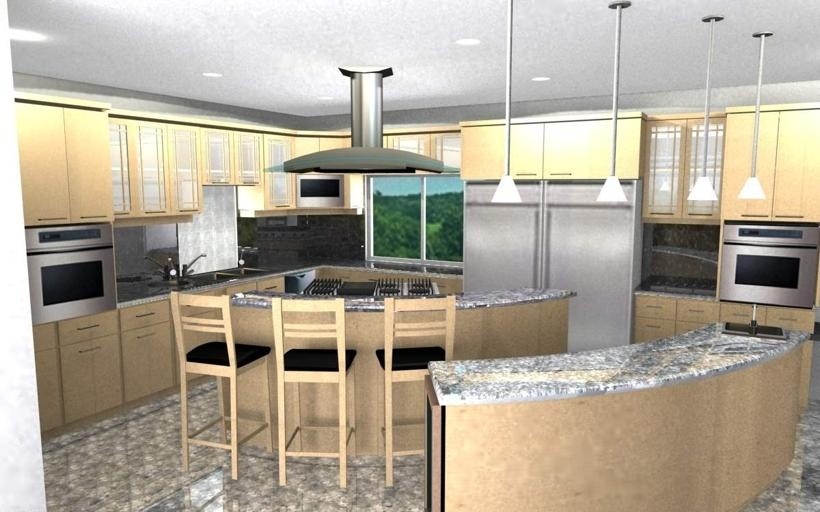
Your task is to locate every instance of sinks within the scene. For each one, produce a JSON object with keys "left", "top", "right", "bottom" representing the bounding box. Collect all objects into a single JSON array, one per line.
[
  {"left": 198, "top": 271, "right": 238, "bottom": 281},
  {"left": 225, "top": 268, "right": 268, "bottom": 276},
  {"left": 116, "top": 276, "right": 152, "bottom": 283},
  {"left": 721, "top": 321, "right": 787, "bottom": 340},
  {"left": 148, "top": 280, "right": 190, "bottom": 288}
]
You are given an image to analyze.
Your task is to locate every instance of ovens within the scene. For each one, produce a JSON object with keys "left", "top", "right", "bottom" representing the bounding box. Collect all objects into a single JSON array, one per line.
[
  {"left": 295, "top": 174, "right": 344, "bottom": 207},
  {"left": 717, "top": 243, "right": 818, "bottom": 310},
  {"left": 25, "top": 244, "right": 119, "bottom": 326}
]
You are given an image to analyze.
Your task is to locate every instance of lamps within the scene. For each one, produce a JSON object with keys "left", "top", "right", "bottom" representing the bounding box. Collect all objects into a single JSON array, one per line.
[
  {"left": 487, "top": 0, "right": 527, "bottom": 205},
  {"left": 686, "top": 14, "right": 726, "bottom": 204},
  {"left": 736, "top": 29, "right": 777, "bottom": 203},
  {"left": 593, "top": 0, "right": 634, "bottom": 208}
]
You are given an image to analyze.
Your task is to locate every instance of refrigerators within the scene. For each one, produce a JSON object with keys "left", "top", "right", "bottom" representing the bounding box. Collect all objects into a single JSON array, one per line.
[{"left": 461, "top": 182, "right": 645, "bottom": 351}]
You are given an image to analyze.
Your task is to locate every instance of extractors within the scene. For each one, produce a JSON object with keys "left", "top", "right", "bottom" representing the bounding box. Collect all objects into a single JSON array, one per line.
[{"left": 259, "top": 63, "right": 460, "bottom": 175}]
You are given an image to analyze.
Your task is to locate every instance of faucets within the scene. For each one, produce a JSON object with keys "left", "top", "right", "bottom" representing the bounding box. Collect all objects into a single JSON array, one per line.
[
  {"left": 748, "top": 303, "right": 759, "bottom": 335},
  {"left": 143, "top": 256, "right": 165, "bottom": 270},
  {"left": 182, "top": 254, "right": 207, "bottom": 277}
]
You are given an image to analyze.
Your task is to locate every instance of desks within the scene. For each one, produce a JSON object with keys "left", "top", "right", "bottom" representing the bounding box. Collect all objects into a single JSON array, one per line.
[{"left": 220, "top": 286, "right": 578, "bottom": 458}]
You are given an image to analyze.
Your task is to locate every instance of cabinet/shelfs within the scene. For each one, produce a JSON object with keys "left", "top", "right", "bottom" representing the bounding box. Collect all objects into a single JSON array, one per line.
[
  {"left": 14, "top": 95, "right": 114, "bottom": 226},
  {"left": 227, "top": 273, "right": 284, "bottom": 299},
  {"left": 642, "top": 113, "right": 723, "bottom": 225},
  {"left": 719, "top": 300, "right": 815, "bottom": 410},
  {"left": 632, "top": 294, "right": 720, "bottom": 346},
  {"left": 175, "top": 284, "right": 228, "bottom": 386},
  {"left": 32, "top": 322, "right": 66, "bottom": 435},
  {"left": 57, "top": 297, "right": 176, "bottom": 426},
  {"left": 320, "top": 267, "right": 463, "bottom": 297},
  {"left": 720, "top": 109, "right": 820, "bottom": 224}
]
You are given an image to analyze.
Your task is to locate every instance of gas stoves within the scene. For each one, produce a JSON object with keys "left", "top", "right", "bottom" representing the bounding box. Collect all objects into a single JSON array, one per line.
[{"left": 302, "top": 276, "right": 434, "bottom": 297}]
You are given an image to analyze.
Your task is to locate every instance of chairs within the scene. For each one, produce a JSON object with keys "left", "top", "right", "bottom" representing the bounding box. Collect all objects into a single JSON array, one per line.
[
  {"left": 373, "top": 292, "right": 458, "bottom": 489},
  {"left": 168, "top": 290, "right": 274, "bottom": 481},
  {"left": 269, "top": 293, "right": 360, "bottom": 495}
]
